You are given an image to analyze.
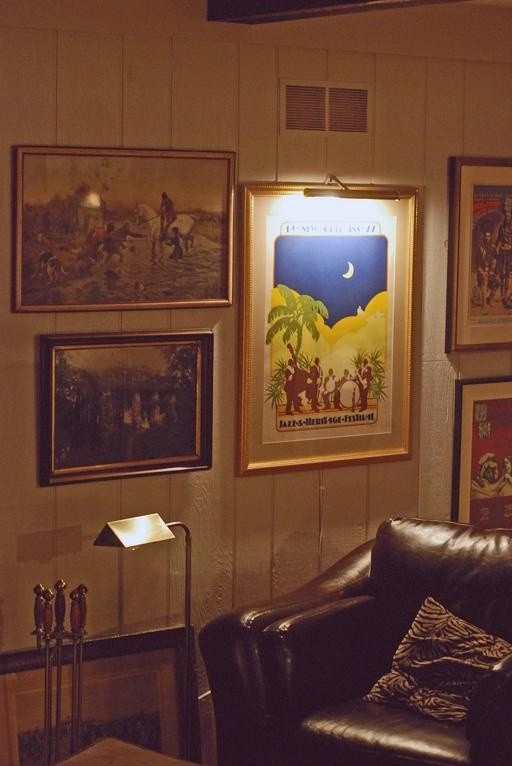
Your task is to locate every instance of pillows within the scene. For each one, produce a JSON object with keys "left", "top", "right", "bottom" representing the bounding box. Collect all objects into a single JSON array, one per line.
[{"left": 362, "top": 595, "right": 512, "bottom": 725}]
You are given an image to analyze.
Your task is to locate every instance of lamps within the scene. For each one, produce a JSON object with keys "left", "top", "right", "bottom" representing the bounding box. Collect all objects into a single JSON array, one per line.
[
  {"left": 304, "top": 174, "right": 400, "bottom": 200},
  {"left": 93, "top": 512, "right": 192, "bottom": 758}
]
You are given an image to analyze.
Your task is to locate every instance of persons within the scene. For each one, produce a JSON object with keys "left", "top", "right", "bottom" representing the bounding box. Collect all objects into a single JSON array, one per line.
[
  {"left": 476, "top": 206, "right": 512, "bottom": 314},
  {"left": 33, "top": 191, "right": 194, "bottom": 288},
  {"left": 284, "top": 359, "right": 372, "bottom": 415}
]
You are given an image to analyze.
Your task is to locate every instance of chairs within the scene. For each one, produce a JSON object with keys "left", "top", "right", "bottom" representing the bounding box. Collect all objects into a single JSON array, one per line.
[{"left": 197, "top": 516, "right": 512, "bottom": 766}]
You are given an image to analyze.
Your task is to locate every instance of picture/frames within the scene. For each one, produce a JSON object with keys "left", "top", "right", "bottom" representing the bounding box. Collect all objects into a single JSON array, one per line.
[
  {"left": 445, "top": 155, "right": 512, "bottom": 352},
  {"left": 451, "top": 376, "right": 512, "bottom": 533},
  {"left": 11, "top": 144, "right": 236, "bottom": 313},
  {"left": 234, "top": 181, "right": 425, "bottom": 478},
  {"left": 35, "top": 328, "right": 214, "bottom": 487},
  {"left": 0, "top": 625, "right": 201, "bottom": 766}
]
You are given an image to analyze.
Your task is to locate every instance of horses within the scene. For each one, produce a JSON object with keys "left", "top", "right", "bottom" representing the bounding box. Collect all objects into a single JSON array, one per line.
[{"left": 133, "top": 201, "right": 199, "bottom": 262}]
